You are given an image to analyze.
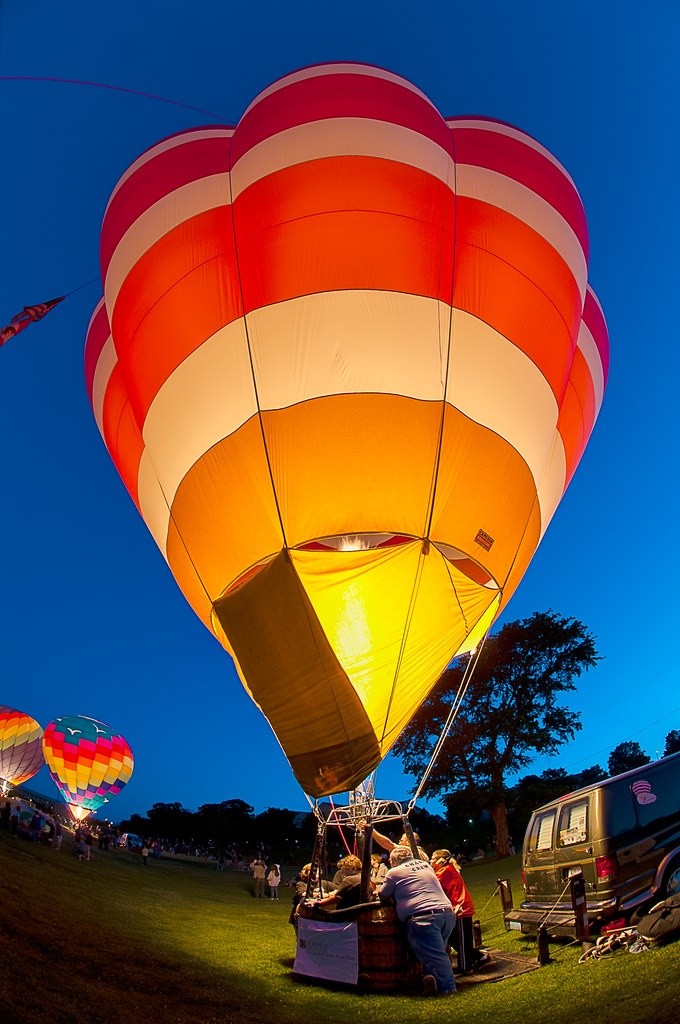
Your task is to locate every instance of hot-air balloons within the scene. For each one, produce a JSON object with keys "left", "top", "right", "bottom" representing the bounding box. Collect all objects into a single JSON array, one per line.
[
  {"left": 82, "top": 61, "right": 614, "bottom": 988},
  {"left": 0, "top": 704, "right": 45, "bottom": 812},
  {"left": 41, "top": 715, "right": 135, "bottom": 864}
]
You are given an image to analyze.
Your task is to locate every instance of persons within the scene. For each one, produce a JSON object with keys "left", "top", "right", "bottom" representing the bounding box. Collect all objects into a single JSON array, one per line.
[
  {"left": 0, "top": 799, "right": 489, "bottom": 974},
  {"left": 378, "top": 845, "right": 459, "bottom": 996}
]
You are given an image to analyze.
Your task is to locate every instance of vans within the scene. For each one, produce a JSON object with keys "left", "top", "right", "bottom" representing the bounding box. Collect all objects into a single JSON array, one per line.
[
  {"left": 503, "top": 752, "right": 678, "bottom": 937},
  {"left": 119, "top": 833, "right": 143, "bottom": 850}
]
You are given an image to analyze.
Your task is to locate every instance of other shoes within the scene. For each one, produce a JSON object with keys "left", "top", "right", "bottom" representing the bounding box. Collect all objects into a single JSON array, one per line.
[
  {"left": 472, "top": 954, "right": 491, "bottom": 969},
  {"left": 422, "top": 974, "right": 438, "bottom": 997},
  {"left": 442, "top": 988, "right": 458, "bottom": 994},
  {"left": 254, "top": 896, "right": 259, "bottom": 899},
  {"left": 453, "top": 966, "right": 474, "bottom": 975},
  {"left": 259, "top": 896, "right": 264, "bottom": 899},
  {"left": 274, "top": 898, "right": 280, "bottom": 901},
  {"left": 269, "top": 897, "right": 275, "bottom": 901}
]
